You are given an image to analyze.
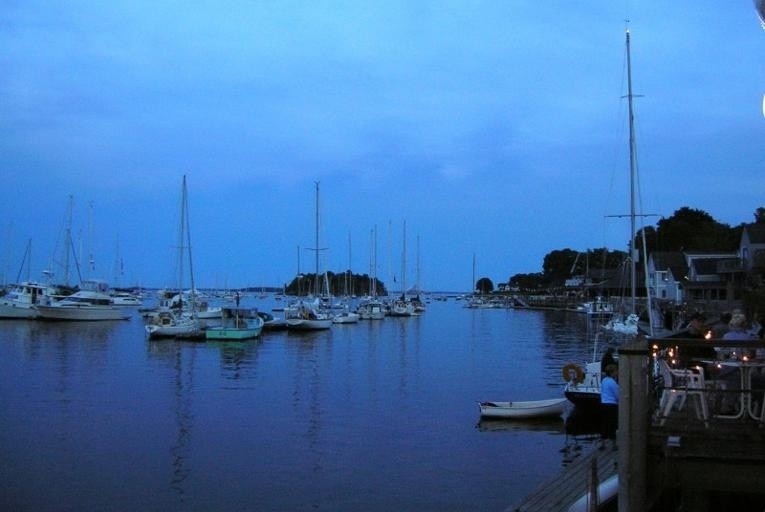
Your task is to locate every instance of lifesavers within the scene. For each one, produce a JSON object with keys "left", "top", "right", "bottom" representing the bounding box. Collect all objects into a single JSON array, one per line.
[{"left": 563, "top": 364, "right": 583, "bottom": 383}]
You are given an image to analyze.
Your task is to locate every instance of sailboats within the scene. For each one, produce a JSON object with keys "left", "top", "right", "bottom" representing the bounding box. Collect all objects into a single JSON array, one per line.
[
  {"left": 561, "top": 23, "right": 664, "bottom": 409},
  {"left": 1, "top": 195, "right": 142, "bottom": 323}
]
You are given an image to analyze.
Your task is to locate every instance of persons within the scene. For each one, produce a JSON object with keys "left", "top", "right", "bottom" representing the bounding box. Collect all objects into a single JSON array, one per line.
[
  {"left": 601, "top": 346, "right": 618, "bottom": 371},
  {"left": 598, "top": 364, "right": 621, "bottom": 452},
  {"left": 720, "top": 312, "right": 765, "bottom": 362},
  {"left": 673, "top": 311, "right": 718, "bottom": 359}
]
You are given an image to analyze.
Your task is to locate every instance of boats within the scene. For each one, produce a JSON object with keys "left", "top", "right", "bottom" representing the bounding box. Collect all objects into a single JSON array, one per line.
[
  {"left": 476, "top": 395, "right": 568, "bottom": 419},
  {"left": 587, "top": 294, "right": 619, "bottom": 321}
]
